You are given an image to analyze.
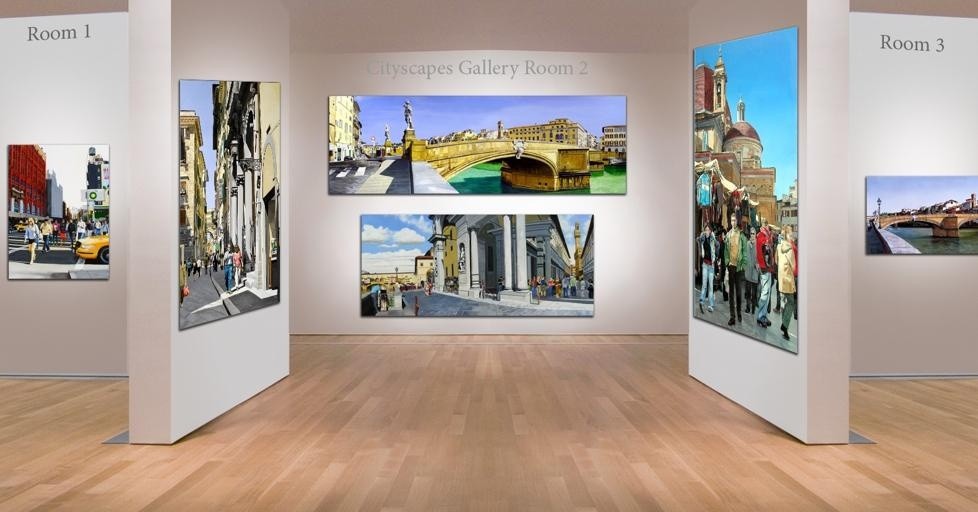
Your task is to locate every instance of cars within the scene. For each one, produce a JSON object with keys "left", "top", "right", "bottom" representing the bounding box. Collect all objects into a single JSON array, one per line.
[
  {"left": 76, "top": 233, "right": 109, "bottom": 264},
  {"left": 13, "top": 220, "right": 29, "bottom": 232}
]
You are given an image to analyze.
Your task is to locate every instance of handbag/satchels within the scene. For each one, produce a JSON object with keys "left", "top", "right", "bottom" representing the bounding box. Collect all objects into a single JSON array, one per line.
[{"left": 756, "top": 232, "right": 769, "bottom": 274}]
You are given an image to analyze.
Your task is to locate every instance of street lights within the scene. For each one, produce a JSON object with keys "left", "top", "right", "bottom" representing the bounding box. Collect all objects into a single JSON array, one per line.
[{"left": 877, "top": 198, "right": 882, "bottom": 229}]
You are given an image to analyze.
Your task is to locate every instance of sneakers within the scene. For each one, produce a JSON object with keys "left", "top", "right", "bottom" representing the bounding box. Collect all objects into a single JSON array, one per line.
[{"left": 698, "top": 291, "right": 792, "bottom": 341}]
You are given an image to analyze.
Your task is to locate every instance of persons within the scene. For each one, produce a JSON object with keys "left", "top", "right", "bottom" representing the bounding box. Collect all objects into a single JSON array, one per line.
[
  {"left": 180, "top": 243, "right": 243, "bottom": 308},
  {"left": 390, "top": 282, "right": 433, "bottom": 297},
  {"left": 23, "top": 214, "right": 110, "bottom": 265},
  {"left": 694, "top": 211, "right": 798, "bottom": 341},
  {"left": 528, "top": 272, "right": 594, "bottom": 300},
  {"left": 385, "top": 124, "right": 391, "bottom": 140},
  {"left": 403, "top": 98, "right": 414, "bottom": 130}
]
